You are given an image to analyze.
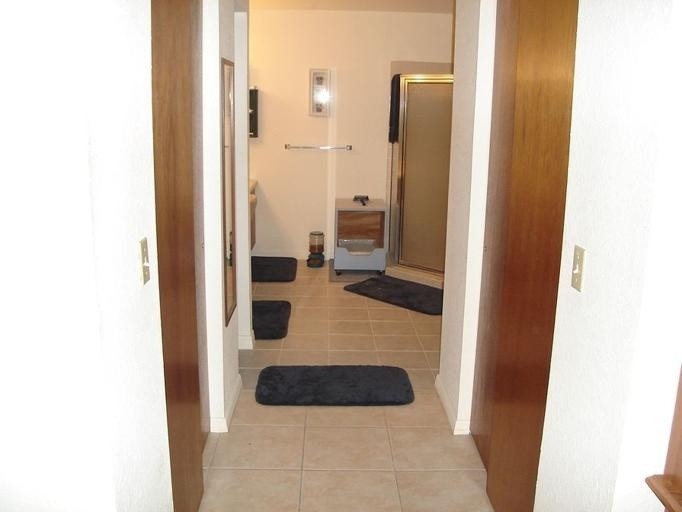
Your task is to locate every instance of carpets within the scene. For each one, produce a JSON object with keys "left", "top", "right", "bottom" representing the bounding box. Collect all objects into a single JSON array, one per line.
[
  {"left": 328, "top": 257, "right": 381, "bottom": 282},
  {"left": 344, "top": 275, "right": 443, "bottom": 317},
  {"left": 256, "top": 364, "right": 415, "bottom": 405},
  {"left": 251, "top": 254, "right": 297, "bottom": 282},
  {"left": 252, "top": 299, "right": 291, "bottom": 340}
]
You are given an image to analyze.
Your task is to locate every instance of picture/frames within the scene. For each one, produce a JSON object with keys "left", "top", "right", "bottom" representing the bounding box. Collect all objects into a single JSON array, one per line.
[{"left": 308, "top": 68, "right": 330, "bottom": 118}]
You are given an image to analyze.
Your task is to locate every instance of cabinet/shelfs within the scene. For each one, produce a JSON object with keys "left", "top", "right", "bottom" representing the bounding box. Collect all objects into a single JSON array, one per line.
[{"left": 334, "top": 198, "right": 386, "bottom": 273}]
